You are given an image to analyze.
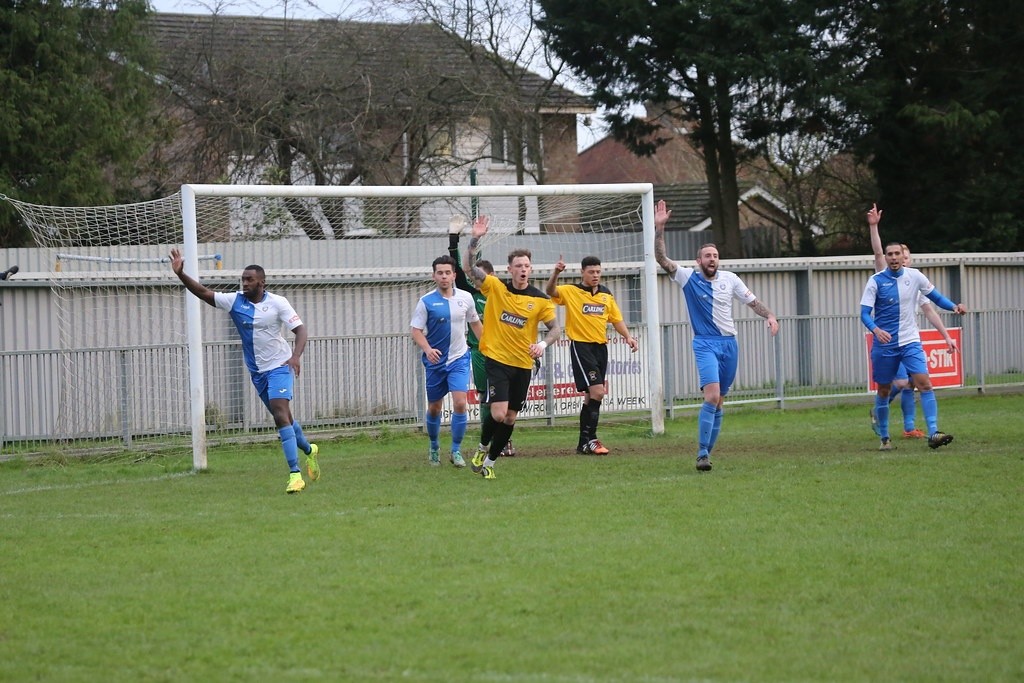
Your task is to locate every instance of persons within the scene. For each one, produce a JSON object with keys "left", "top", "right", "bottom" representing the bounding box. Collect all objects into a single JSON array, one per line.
[
  {"left": 867, "top": 203, "right": 961, "bottom": 437},
  {"left": 410, "top": 256, "right": 483, "bottom": 468},
  {"left": 447, "top": 215, "right": 516, "bottom": 457},
  {"left": 169, "top": 247, "right": 320, "bottom": 493},
  {"left": 547, "top": 254, "right": 640, "bottom": 455},
  {"left": 655, "top": 199, "right": 778, "bottom": 470},
  {"left": 466, "top": 215, "right": 561, "bottom": 479},
  {"left": 860, "top": 243, "right": 967, "bottom": 452}
]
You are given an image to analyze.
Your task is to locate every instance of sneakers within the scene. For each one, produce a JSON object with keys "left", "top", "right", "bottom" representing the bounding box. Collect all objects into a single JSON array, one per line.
[
  {"left": 304, "top": 443, "right": 321, "bottom": 482},
  {"left": 449, "top": 449, "right": 467, "bottom": 468},
  {"left": 499, "top": 438, "right": 515, "bottom": 457},
  {"left": 696, "top": 456, "right": 712, "bottom": 470},
  {"left": 588, "top": 439, "right": 609, "bottom": 455},
  {"left": 869, "top": 408, "right": 882, "bottom": 436},
  {"left": 577, "top": 443, "right": 592, "bottom": 455},
  {"left": 903, "top": 428, "right": 929, "bottom": 439},
  {"left": 878, "top": 439, "right": 893, "bottom": 451},
  {"left": 927, "top": 431, "right": 953, "bottom": 449},
  {"left": 429, "top": 447, "right": 441, "bottom": 468},
  {"left": 470, "top": 447, "right": 488, "bottom": 475},
  {"left": 286, "top": 471, "right": 306, "bottom": 494},
  {"left": 480, "top": 465, "right": 496, "bottom": 480}
]
